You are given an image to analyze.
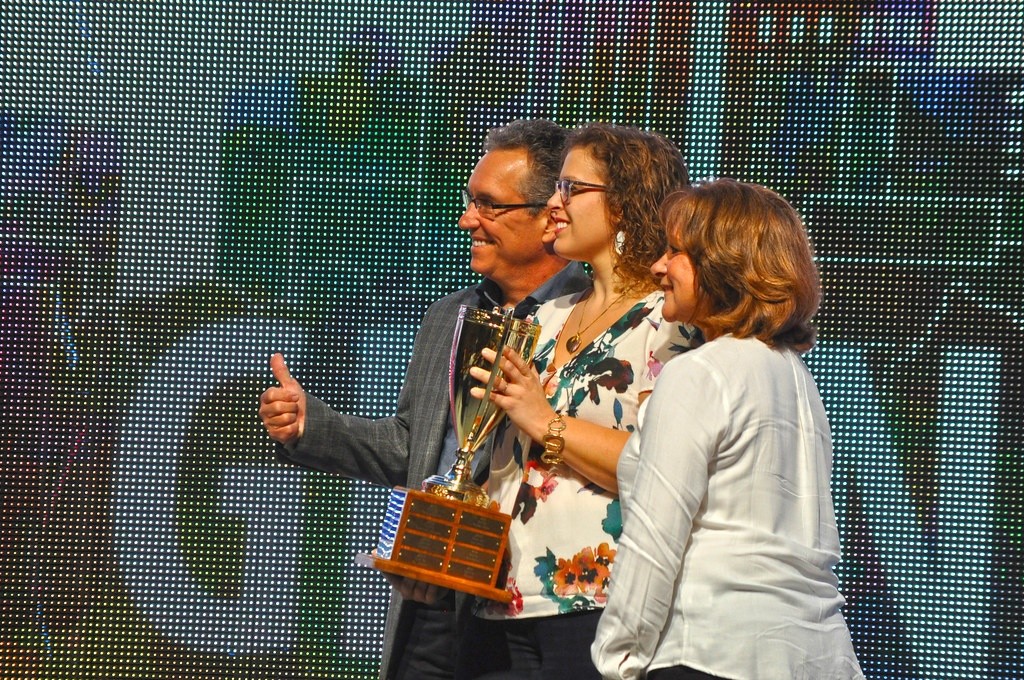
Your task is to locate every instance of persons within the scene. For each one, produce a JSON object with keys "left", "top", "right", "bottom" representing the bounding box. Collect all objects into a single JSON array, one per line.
[
  {"left": 257, "top": 122, "right": 704, "bottom": 680},
  {"left": 588, "top": 180, "right": 871, "bottom": 680}
]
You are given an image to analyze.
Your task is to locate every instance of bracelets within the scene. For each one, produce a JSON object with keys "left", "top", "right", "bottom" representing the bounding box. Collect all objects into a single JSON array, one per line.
[{"left": 541, "top": 412, "right": 566, "bottom": 465}]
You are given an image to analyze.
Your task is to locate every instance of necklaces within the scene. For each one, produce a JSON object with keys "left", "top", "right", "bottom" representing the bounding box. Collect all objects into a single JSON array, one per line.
[{"left": 565, "top": 281, "right": 637, "bottom": 354}]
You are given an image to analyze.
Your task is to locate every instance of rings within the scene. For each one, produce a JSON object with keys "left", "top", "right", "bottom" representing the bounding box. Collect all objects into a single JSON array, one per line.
[{"left": 501, "top": 381, "right": 509, "bottom": 393}]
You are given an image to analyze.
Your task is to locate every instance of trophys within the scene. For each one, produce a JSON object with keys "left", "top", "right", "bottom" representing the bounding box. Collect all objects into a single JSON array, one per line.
[{"left": 358, "top": 300, "right": 541, "bottom": 601}]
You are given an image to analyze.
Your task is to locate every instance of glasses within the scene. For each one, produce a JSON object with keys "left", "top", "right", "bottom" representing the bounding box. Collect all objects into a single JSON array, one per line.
[
  {"left": 461, "top": 189, "right": 547, "bottom": 221},
  {"left": 555, "top": 179, "right": 610, "bottom": 205}
]
